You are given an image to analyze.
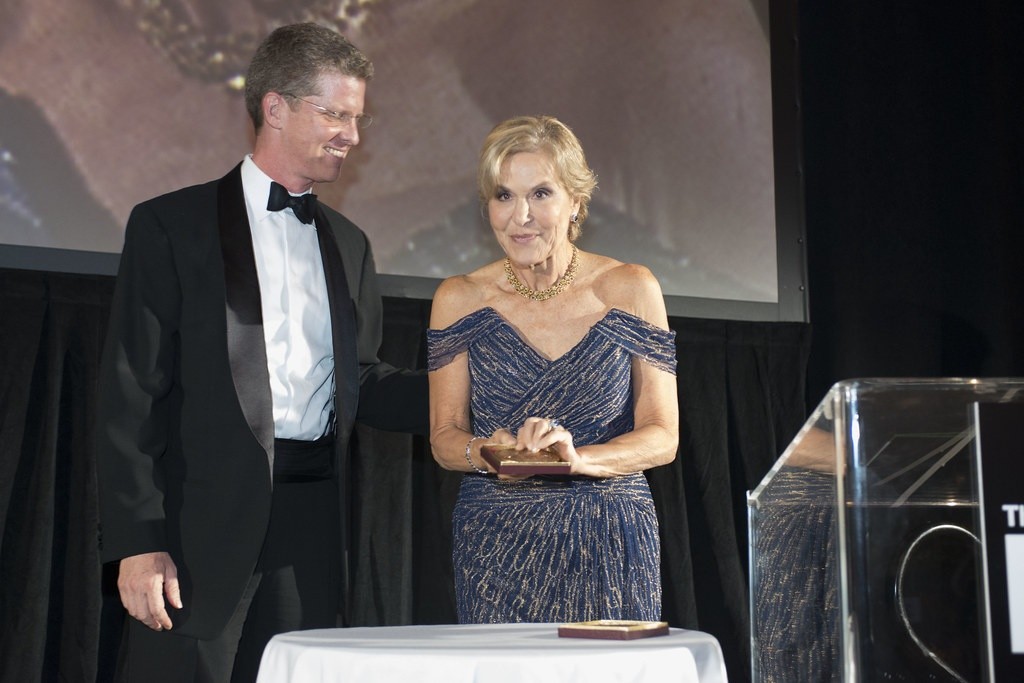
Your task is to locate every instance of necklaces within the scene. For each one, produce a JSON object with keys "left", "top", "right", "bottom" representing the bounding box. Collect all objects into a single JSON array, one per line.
[{"left": 505, "top": 242, "right": 580, "bottom": 299}]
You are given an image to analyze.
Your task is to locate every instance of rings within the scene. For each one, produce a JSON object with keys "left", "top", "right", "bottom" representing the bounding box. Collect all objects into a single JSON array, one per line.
[{"left": 549, "top": 419, "right": 558, "bottom": 431}]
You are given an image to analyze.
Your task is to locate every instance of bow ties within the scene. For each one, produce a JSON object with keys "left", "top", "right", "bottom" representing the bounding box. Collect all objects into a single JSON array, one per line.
[{"left": 266, "top": 181, "right": 317, "bottom": 224}]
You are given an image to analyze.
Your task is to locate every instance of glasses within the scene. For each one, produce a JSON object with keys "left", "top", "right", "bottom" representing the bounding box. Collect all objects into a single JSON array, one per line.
[{"left": 278, "top": 91, "right": 373, "bottom": 129}]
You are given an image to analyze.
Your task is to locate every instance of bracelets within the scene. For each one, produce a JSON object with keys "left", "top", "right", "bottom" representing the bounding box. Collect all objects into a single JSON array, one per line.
[{"left": 466, "top": 436, "right": 490, "bottom": 473}]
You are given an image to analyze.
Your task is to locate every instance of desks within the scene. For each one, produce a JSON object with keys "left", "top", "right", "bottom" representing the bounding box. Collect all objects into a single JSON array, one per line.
[{"left": 255, "top": 623, "right": 728, "bottom": 683}]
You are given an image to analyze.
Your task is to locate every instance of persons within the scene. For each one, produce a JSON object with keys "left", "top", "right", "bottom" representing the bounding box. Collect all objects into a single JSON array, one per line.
[
  {"left": 96, "top": 24, "right": 429, "bottom": 683},
  {"left": 747, "top": 425, "right": 846, "bottom": 683},
  {"left": 429, "top": 115, "right": 679, "bottom": 626}
]
